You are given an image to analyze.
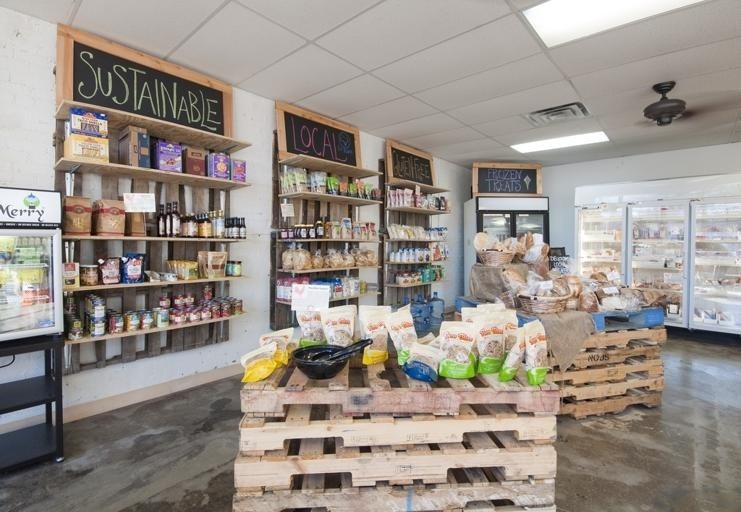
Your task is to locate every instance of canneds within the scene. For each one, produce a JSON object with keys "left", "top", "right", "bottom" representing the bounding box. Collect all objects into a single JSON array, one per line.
[
  {"left": 63, "top": 284, "right": 243, "bottom": 340},
  {"left": 281, "top": 224, "right": 316, "bottom": 239},
  {"left": 226, "top": 260, "right": 242, "bottom": 277}
]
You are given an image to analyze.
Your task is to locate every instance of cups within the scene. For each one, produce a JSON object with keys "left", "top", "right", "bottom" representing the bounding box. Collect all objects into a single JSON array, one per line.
[{"left": 79, "top": 265, "right": 100, "bottom": 286}]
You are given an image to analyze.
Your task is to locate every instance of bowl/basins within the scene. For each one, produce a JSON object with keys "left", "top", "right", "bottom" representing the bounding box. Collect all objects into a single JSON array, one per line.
[{"left": 292, "top": 344, "right": 351, "bottom": 380}]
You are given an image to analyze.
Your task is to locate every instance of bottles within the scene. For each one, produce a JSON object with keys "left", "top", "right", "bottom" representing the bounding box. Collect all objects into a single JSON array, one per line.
[
  {"left": 396, "top": 265, "right": 447, "bottom": 286},
  {"left": 157, "top": 202, "right": 246, "bottom": 240},
  {"left": 388, "top": 246, "right": 431, "bottom": 263},
  {"left": 440, "top": 196, "right": 447, "bottom": 211},
  {"left": 279, "top": 217, "right": 331, "bottom": 238}
]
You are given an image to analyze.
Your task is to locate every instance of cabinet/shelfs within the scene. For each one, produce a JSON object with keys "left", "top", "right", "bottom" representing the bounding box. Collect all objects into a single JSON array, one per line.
[
  {"left": 384, "top": 178, "right": 451, "bottom": 287},
  {"left": 55, "top": 100, "right": 251, "bottom": 345},
  {"left": 274, "top": 153, "right": 384, "bottom": 308},
  {"left": 0, "top": 335, "right": 64, "bottom": 477}
]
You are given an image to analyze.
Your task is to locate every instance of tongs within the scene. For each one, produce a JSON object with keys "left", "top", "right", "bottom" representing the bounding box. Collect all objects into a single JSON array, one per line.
[{"left": 314, "top": 339, "right": 375, "bottom": 362}]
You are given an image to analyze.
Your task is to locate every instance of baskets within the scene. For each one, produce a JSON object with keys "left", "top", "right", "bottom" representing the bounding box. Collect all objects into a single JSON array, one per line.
[
  {"left": 515, "top": 242, "right": 549, "bottom": 264},
  {"left": 474, "top": 232, "right": 515, "bottom": 266},
  {"left": 517, "top": 277, "right": 571, "bottom": 314}
]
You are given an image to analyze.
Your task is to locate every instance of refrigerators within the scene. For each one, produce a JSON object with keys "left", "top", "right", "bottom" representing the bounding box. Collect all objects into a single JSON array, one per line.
[{"left": 463, "top": 195, "right": 551, "bottom": 295}]
